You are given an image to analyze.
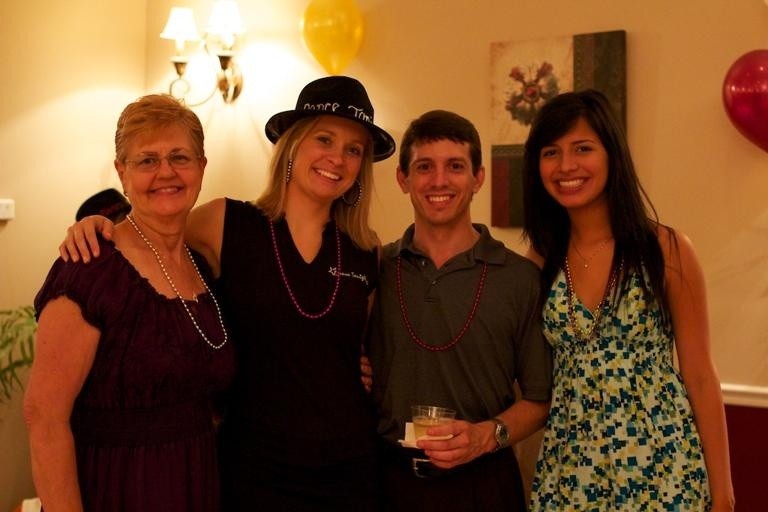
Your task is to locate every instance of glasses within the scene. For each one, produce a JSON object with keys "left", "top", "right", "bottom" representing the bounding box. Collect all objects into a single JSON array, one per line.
[{"left": 121, "top": 149, "right": 198, "bottom": 171}]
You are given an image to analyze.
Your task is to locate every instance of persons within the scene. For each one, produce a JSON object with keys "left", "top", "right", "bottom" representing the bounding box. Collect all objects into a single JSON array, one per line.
[
  {"left": 25, "top": 93, "right": 235, "bottom": 512},
  {"left": 361, "top": 88, "right": 737, "bottom": 512},
  {"left": 367, "top": 112, "right": 552, "bottom": 512},
  {"left": 58, "top": 75, "right": 396, "bottom": 512}
]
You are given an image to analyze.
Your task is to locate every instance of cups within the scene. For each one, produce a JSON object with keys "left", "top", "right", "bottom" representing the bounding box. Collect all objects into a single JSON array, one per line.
[{"left": 410, "top": 404, "right": 456, "bottom": 444}]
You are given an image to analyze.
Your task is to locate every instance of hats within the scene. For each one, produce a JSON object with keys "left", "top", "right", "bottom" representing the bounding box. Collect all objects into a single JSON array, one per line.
[{"left": 265, "top": 75, "right": 397, "bottom": 166}]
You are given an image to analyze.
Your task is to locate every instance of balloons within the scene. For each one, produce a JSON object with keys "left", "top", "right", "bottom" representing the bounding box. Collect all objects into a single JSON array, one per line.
[
  {"left": 300, "top": 0, "right": 363, "bottom": 75},
  {"left": 722, "top": 49, "right": 768, "bottom": 152}
]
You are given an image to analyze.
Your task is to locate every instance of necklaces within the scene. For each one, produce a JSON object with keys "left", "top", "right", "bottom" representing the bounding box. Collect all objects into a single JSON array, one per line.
[
  {"left": 269, "top": 216, "right": 342, "bottom": 319},
  {"left": 125, "top": 213, "right": 228, "bottom": 349},
  {"left": 570, "top": 236, "right": 610, "bottom": 268},
  {"left": 562, "top": 258, "right": 624, "bottom": 345},
  {"left": 396, "top": 254, "right": 488, "bottom": 351}
]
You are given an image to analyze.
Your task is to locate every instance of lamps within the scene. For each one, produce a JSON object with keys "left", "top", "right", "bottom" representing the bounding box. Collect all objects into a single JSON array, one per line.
[{"left": 161, "top": 4, "right": 243, "bottom": 106}]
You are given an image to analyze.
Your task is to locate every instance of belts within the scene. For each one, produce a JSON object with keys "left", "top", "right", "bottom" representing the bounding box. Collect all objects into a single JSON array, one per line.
[{"left": 405, "top": 450, "right": 447, "bottom": 480}]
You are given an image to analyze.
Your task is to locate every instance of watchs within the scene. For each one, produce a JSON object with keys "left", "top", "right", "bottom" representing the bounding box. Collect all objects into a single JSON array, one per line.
[{"left": 492, "top": 418, "right": 509, "bottom": 452}]
